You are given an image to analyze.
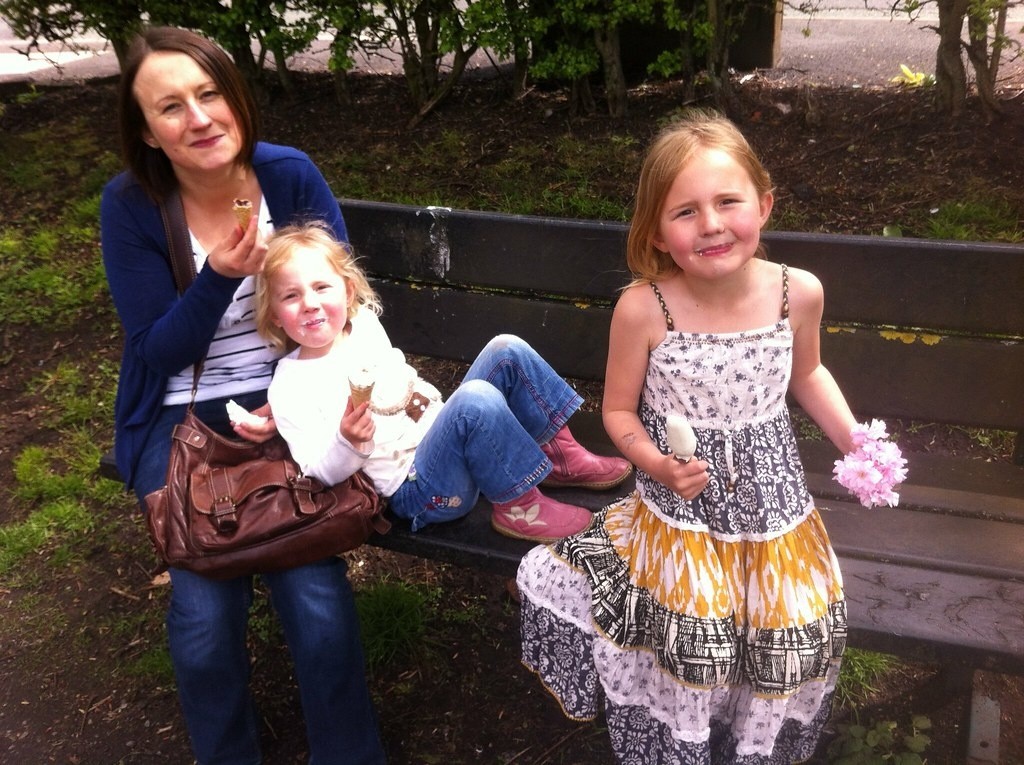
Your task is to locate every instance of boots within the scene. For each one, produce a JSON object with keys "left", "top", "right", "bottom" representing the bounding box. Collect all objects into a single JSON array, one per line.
[
  {"left": 537, "top": 424, "right": 632, "bottom": 490},
  {"left": 491, "top": 487, "right": 595, "bottom": 544}
]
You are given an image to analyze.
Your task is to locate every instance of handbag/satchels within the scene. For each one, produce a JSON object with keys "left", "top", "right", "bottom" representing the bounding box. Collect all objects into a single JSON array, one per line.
[{"left": 144, "top": 412, "right": 391, "bottom": 578}]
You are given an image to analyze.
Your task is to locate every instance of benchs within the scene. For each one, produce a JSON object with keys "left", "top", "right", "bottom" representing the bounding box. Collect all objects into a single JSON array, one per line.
[{"left": 336, "top": 196, "right": 1024, "bottom": 765}]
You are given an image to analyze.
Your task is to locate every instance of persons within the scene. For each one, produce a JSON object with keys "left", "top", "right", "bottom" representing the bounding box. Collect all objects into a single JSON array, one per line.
[
  {"left": 252, "top": 219, "right": 633, "bottom": 544},
  {"left": 99, "top": 22, "right": 386, "bottom": 765},
  {"left": 514, "top": 108, "right": 908, "bottom": 765}
]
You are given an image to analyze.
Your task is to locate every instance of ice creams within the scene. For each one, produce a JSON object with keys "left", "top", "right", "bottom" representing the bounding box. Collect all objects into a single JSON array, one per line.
[
  {"left": 232, "top": 197, "right": 253, "bottom": 235},
  {"left": 347, "top": 367, "right": 376, "bottom": 411}
]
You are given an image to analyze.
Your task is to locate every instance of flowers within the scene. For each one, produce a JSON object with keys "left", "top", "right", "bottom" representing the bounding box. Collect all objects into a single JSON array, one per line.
[{"left": 833, "top": 415, "right": 909, "bottom": 509}]
